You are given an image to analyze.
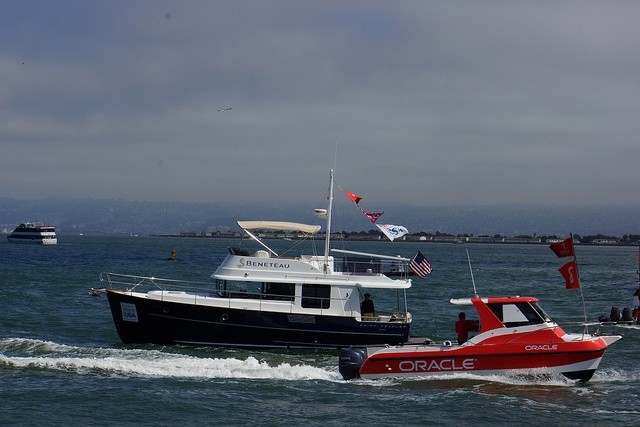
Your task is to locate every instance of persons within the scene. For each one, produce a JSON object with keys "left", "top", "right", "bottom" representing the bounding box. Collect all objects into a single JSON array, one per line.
[
  {"left": 455, "top": 312, "right": 479, "bottom": 345},
  {"left": 360, "top": 292, "right": 375, "bottom": 321}
]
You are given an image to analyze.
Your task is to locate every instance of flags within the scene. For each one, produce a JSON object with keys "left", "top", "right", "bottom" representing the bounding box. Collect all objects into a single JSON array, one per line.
[
  {"left": 344, "top": 192, "right": 364, "bottom": 204},
  {"left": 558, "top": 259, "right": 579, "bottom": 289},
  {"left": 376, "top": 222, "right": 409, "bottom": 242},
  {"left": 549, "top": 238, "right": 575, "bottom": 259},
  {"left": 408, "top": 251, "right": 433, "bottom": 279},
  {"left": 357, "top": 206, "right": 384, "bottom": 223}
]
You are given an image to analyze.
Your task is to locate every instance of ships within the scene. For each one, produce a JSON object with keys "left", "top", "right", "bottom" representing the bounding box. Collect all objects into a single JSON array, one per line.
[{"left": 7, "top": 223, "right": 58, "bottom": 244}]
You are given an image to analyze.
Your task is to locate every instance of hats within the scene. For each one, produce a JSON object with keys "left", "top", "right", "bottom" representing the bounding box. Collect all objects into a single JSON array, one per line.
[{"left": 364, "top": 293, "right": 371, "bottom": 296}]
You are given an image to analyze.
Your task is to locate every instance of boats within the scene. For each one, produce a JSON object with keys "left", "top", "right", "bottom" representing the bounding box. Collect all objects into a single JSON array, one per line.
[
  {"left": 99, "top": 168, "right": 412, "bottom": 347},
  {"left": 359, "top": 247, "right": 622, "bottom": 385}
]
